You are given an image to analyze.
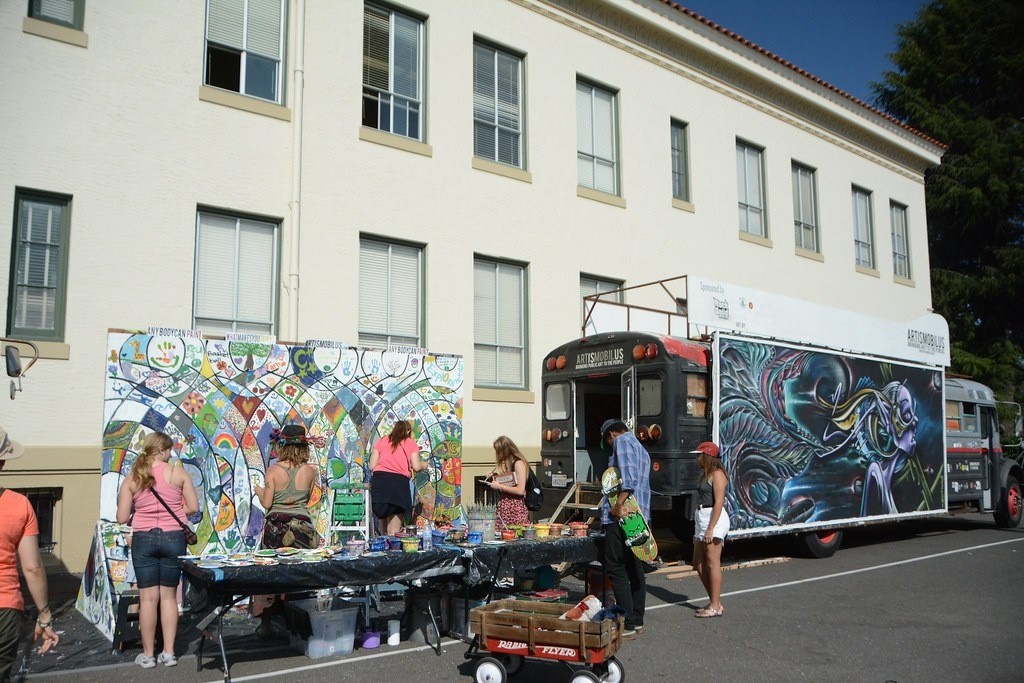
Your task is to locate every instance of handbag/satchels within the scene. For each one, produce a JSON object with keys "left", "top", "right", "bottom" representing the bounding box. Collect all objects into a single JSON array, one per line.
[{"left": 183, "top": 525, "right": 198, "bottom": 545}]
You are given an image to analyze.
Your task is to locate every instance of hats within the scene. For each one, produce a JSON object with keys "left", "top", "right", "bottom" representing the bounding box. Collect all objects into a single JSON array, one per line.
[
  {"left": 270, "top": 425, "right": 325, "bottom": 448},
  {"left": 600, "top": 418, "right": 624, "bottom": 449},
  {"left": 688, "top": 441, "right": 719, "bottom": 459},
  {"left": 0, "top": 427, "right": 24, "bottom": 459}
]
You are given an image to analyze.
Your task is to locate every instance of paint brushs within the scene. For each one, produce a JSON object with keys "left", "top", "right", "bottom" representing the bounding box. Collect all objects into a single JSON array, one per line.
[{"left": 484, "top": 467, "right": 496, "bottom": 481}]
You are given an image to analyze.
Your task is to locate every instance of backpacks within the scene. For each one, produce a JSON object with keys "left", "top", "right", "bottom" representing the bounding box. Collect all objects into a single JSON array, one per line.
[{"left": 512, "top": 461, "right": 543, "bottom": 512}]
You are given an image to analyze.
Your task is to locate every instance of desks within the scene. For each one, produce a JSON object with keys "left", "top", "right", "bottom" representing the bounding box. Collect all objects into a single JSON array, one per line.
[
  {"left": 440, "top": 530, "right": 606, "bottom": 644},
  {"left": 175, "top": 541, "right": 466, "bottom": 683}
]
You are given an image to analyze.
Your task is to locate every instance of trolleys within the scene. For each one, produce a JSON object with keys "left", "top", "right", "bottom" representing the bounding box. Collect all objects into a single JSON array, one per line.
[{"left": 464, "top": 547, "right": 625, "bottom": 683}]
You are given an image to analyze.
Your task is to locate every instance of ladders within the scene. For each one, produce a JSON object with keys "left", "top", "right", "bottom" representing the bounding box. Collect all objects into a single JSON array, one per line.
[{"left": 317, "top": 482, "right": 380, "bottom": 608}]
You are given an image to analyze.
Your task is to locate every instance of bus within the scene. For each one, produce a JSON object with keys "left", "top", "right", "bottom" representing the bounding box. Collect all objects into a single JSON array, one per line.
[{"left": 539, "top": 331, "right": 1024, "bottom": 559}]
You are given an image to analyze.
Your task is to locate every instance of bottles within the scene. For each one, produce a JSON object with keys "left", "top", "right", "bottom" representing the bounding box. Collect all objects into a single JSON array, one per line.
[{"left": 423, "top": 520, "right": 432, "bottom": 552}]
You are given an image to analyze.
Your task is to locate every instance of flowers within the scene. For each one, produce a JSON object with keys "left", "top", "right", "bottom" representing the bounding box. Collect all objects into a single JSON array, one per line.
[{"left": 267, "top": 428, "right": 303, "bottom": 447}]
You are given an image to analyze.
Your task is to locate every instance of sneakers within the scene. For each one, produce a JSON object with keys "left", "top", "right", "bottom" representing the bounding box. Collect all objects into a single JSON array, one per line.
[
  {"left": 135, "top": 653, "right": 156, "bottom": 669},
  {"left": 157, "top": 653, "right": 178, "bottom": 666}
]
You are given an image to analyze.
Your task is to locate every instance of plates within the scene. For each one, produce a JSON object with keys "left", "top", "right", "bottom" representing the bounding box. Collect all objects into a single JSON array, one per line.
[
  {"left": 484, "top": 540, "right": 505, "bottom": 544},
  {"left": 177, "top": 547, "right": 387, "bottom": 568}
]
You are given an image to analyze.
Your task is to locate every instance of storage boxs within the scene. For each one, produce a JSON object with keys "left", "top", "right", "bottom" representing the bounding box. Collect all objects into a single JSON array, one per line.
[
  {"left": 283, "top": 594, "right": 359, "bottom": 659},
  {"left": 452, "top": 586, "right": 624, "bottom": 647}
]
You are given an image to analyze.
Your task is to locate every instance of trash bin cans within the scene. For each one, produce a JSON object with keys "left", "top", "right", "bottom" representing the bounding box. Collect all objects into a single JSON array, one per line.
[{"left": 404, "top": 588, "right": 442, "bottom": 643}]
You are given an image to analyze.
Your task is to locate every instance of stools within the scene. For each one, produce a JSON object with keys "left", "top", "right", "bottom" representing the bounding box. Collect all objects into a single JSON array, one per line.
[
  {"left": 586, "top": 564, "right": 617, "bottom": 605},
  {"left": 110, "top": 590, "right": 164, "bottom": 656}
]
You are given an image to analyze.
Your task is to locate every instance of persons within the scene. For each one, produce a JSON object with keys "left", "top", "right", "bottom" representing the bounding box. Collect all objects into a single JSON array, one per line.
[
  {"left": 253, "top": 425, "right": 315, "bottom": 550},
  {"left": 118, "top": 432, "right": 199, "bottom": 668},
  {"left": 600, "top": 420, "right": 651, "bottom": 640},
  {"left": 485, "top": 436, "right": 528, "bottom": 532},
  {"left": 689, "top": 442, "right": 730, "bottom": 618},
  {"left": 370, "top": 420, "right": 428, "bottom": 536},
  {"left": 0, "top": 427, "right": 58, "bottom": 682}
]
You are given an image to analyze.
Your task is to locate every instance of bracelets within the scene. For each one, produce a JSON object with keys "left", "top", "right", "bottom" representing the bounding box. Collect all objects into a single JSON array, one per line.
[
  {"left": 37, "top": 616, "right": 53, "bottom": 629},
  {"left": 42, "top": 605, "right": 50, "bottom": 612}
]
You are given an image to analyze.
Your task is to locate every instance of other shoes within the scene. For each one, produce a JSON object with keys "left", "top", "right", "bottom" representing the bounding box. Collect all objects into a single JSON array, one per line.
[
  {"left": 622, "top": 628, "right": 637, "bottom": 641},
  {"left": 248, "top": 611, "right": 260, "bottom": 626},
  {"left": 634, "top": 624, "right": 643, "bottom": 635}
]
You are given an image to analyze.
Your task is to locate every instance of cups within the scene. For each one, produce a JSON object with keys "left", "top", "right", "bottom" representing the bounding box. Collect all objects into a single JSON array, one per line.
[{"left": 387, "top": 620, "right": 400, "bottom": 645}]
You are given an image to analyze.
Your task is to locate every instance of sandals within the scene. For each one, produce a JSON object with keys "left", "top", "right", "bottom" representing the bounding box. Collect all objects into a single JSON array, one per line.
[{"left": 696, "top": 602, "right": 722, "bottom": 618}]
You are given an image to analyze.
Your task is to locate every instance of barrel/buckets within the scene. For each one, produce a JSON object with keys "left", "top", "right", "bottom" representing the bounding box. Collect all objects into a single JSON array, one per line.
[
  {"left": 403, "top": 588, "right": 441, "bottom": 643},
  {"left": 362, "top": 629, "right": 380, "bottom": 649}
]
